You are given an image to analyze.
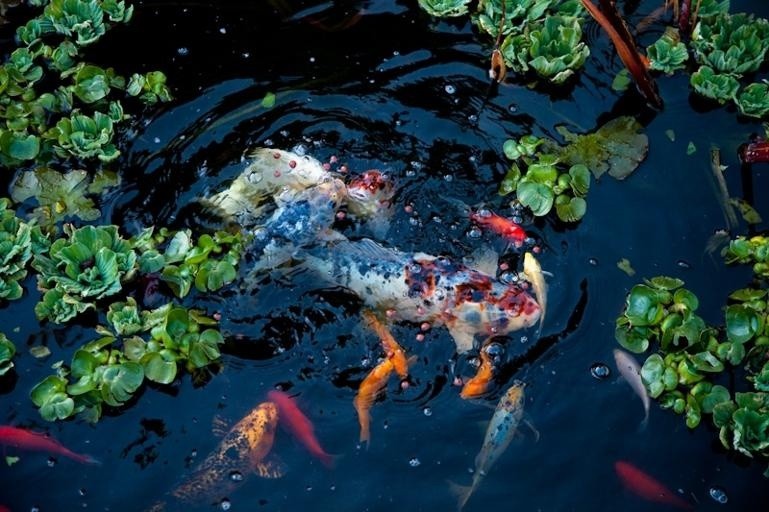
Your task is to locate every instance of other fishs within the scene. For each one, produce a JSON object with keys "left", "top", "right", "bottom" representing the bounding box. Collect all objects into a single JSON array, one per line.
[
  {"left": 147, "top": 148, "right": 688, "bottom": 510},
  {"left": 0, "top": 425, "right": 102, "bottom": 465}
]
